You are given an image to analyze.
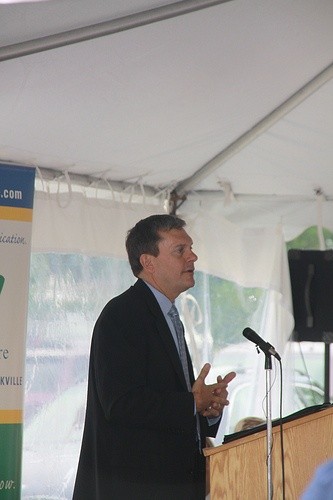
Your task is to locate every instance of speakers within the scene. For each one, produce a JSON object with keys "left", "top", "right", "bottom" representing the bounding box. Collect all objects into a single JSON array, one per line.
[{"left": 287, "top": 249, "right": 333, "bottom": 344}]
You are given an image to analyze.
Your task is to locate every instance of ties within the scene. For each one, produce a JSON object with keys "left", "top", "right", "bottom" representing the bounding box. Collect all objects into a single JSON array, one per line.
[{"left": 168, "top": 305, "right": 201, "bottom": 455}]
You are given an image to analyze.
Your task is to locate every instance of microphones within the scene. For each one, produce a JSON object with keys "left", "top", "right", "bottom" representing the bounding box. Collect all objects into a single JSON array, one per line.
[{"left": 242, "top": 327, "right": 281, "bottom": 360}]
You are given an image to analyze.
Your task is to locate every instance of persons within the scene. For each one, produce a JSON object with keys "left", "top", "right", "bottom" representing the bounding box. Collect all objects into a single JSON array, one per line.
[
  {"left": 234, "top": 417, "right": 265, "bottom": 432},
  {"left": 72, "top": 215, "right": 237, "bottom": 500}
]
